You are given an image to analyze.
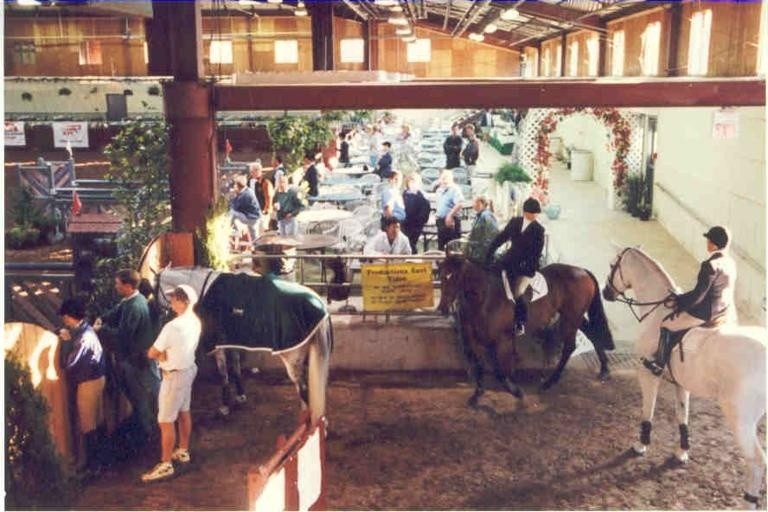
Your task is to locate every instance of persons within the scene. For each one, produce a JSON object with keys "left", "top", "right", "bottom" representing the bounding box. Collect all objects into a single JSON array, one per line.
[
  {"left": 229, "top": 123, "right": 498, "bottom": 312},
  {"left": 640, "top": 225, "right": 736, "bottom": 376},
  {"left": 59, "top": 267, "right": 203, "bottom": 481},
  {"left": 486, "top": 197, "right": 546, "bottom": 337}
]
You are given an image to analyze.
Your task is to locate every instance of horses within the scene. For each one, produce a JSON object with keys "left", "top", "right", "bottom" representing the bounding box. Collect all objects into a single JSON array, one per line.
[
  {"left": 147, "top": 262, "right": 335, "bottom": 440},
  {"left": 433, "top": 246, "right": 619, "bottom": 404},
  {"left": 604, "top": 240, "right": 767, "bottom": 510}
]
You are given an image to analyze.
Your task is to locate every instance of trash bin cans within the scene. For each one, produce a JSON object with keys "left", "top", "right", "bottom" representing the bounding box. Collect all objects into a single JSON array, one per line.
[
  {"left": 571, "top": 149, "right": 594, "bottom": 183},
  {"left": 548, "top": 136, "right": 561, "bottom": 154}
]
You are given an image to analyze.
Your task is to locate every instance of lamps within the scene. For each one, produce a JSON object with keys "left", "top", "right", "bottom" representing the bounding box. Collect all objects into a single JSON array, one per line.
[{"left": 363, "top": 0, "right": 528, "bottom": 42}]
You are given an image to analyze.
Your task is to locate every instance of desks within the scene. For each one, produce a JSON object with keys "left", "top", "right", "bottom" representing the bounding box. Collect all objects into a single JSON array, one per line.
[{"left": 250, "top": 123, "right": 494, "bottom": 312}]
